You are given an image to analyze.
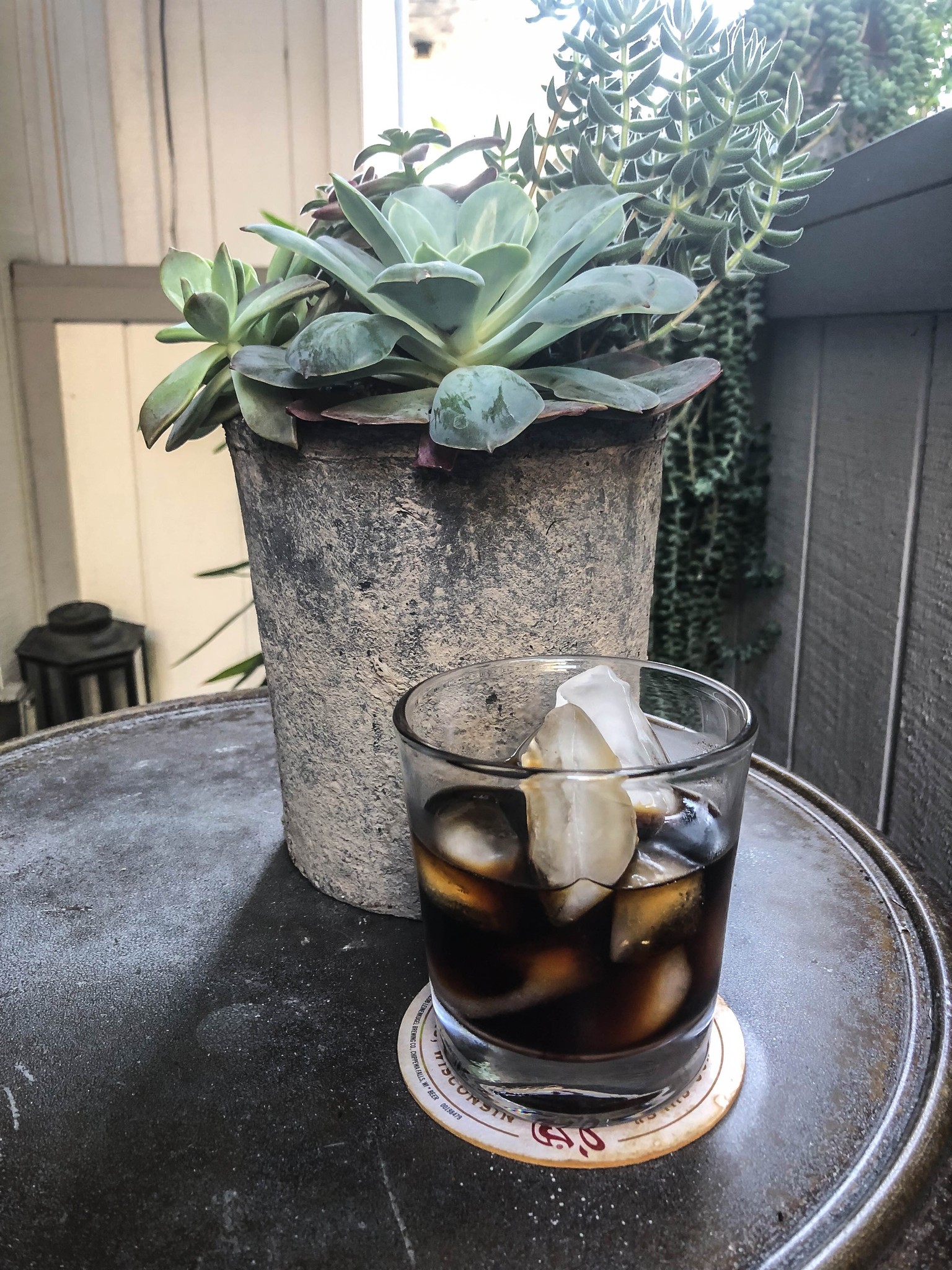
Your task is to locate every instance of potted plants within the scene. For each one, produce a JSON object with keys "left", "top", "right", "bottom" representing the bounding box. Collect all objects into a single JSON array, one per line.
[{"left": 134, "top": 0, "right": 841, "bottom": 923}]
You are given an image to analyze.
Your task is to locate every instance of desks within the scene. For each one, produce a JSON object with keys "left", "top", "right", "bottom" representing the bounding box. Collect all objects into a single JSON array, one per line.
[{"left": 0, "top": 684, "right": 952, "bottom": 1270}]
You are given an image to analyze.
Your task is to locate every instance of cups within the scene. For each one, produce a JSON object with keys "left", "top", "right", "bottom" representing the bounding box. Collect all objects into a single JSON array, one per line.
[{"left": 392, "top": 654, "right": 761, "bottom": 1129}]
